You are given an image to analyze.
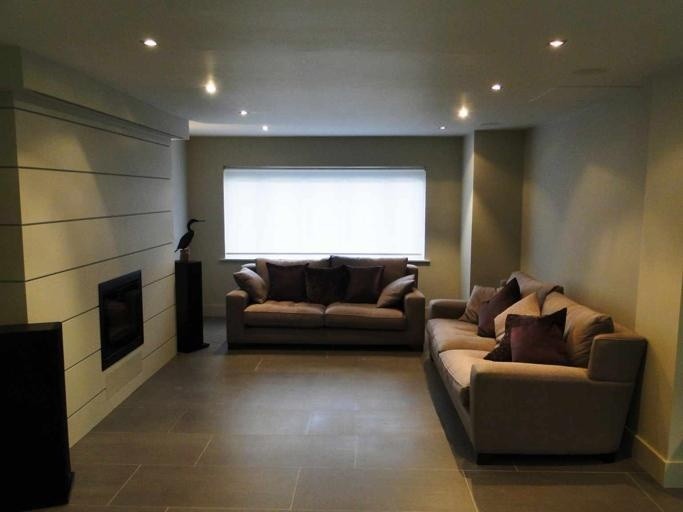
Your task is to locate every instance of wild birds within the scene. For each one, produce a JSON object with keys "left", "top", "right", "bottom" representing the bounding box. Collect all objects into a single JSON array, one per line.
[{"left": 174, "top": 218, "right": 205, "bottom": 253}]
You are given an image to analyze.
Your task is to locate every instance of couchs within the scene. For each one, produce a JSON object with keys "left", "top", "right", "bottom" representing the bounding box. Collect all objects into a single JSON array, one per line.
[
  {"left": 427, "top": 271, "right": 648, "bottom": 465},
  {"left": 226, "top": 264, "right": 425, "bottom": 351}
]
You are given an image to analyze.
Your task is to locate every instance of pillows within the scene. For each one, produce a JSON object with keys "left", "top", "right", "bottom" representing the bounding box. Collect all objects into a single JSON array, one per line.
[
  {"left": 459, "top": 271, "right": 615, "bottom": 368},
  {"left": 233, "top": 256, "right": 417, "bottom": 307}
]
